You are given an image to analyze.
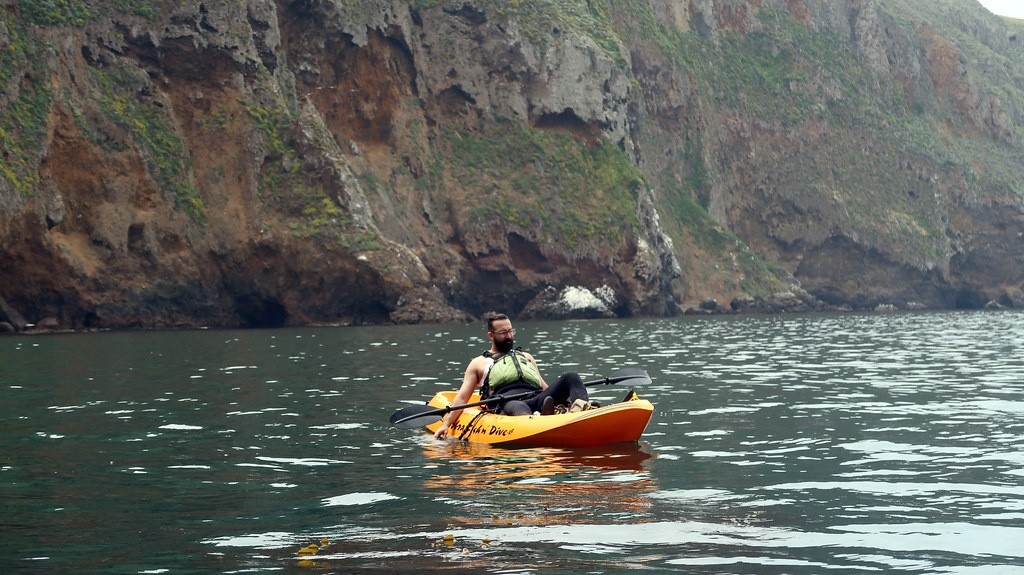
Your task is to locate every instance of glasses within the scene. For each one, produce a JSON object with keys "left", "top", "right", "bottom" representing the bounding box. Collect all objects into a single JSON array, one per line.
[{"left": 493, "top": 328, "right": 517, "bottom": 336}]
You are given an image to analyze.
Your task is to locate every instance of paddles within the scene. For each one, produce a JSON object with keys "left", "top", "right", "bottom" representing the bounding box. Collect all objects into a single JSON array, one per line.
[{"left": 389, "top": 366, "right": 654, "bottom": 430}]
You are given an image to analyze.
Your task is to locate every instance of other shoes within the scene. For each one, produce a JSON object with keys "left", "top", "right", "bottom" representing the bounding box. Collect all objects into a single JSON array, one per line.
[{"left": 542, "top": 396, "right": 554, "bottom": 415}]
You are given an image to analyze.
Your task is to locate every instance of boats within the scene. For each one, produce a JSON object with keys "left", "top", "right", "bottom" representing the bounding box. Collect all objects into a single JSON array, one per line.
[{"left": 425, "top": 390, "right": 655, "bottom": 448}]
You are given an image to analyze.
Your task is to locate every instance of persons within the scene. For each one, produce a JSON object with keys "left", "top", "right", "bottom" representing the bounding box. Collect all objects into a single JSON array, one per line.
[{"left": 434, "top": 314, "right": 590, "bottom": 442}]
